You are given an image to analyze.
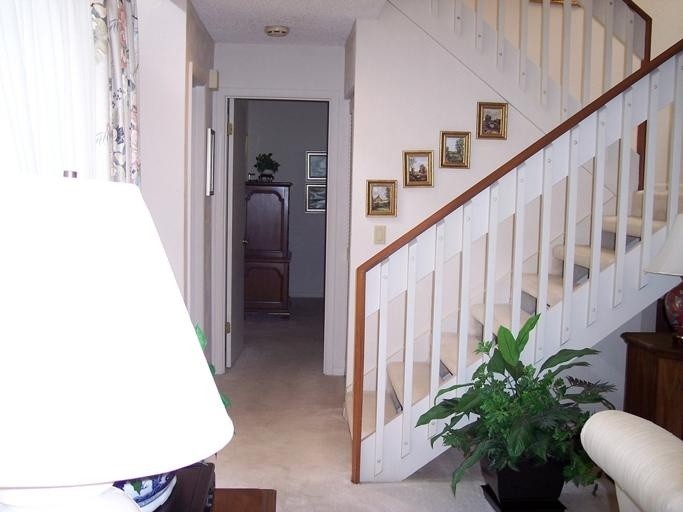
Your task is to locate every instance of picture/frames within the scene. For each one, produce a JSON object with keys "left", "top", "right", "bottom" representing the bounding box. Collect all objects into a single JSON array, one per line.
[
  {"left": 401, "top": 150, "right": 434, "bottom": 188},
  {"left": 439, "top": 130, "right": 471, "bottom": 169},
  {"left": 206, "top": 128, "right": 215, "bottom": 197},
  {"left": 305, "top": 151, "right": 327, "bottom": 213},
  {"left": 365, "top": 179, "right": 397, "bottom": 217},
  {"left": 476, "top": 101, "right": 508, "bottom": 140}
]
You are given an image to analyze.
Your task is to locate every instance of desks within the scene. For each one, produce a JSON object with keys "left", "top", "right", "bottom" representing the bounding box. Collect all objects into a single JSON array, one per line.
[
  {"left": 213, "top": 489, "right": 276, "bottom": 511},
  {"left": 152, "top": 462, "right": 215, "bottom": 510},
  {"left": 620, "top": 331, "right": 682, "bottom": 441}
]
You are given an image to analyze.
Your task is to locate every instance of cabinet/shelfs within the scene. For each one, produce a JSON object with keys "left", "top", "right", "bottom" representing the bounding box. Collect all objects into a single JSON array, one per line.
[{"left": 243, "top": 182, "right": 292, "bottom": 318}]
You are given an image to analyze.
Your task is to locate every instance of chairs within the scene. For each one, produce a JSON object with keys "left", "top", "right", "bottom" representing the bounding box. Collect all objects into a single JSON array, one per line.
[{"left": 579, "top": 410, "right": 683, "bottom": 511}]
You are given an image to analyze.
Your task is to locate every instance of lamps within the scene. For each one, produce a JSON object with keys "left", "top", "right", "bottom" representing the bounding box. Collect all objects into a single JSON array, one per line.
[
  {"left": 643, "top": 213, "right": 682, "bottom": 340},
  {"left": 5, "top": 178, "right": 235, "bottom": 490}
]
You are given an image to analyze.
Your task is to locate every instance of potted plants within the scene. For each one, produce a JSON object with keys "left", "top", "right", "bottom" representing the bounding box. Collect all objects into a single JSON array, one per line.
[
  {"left": 414, "top": 312, "right": 622, "bottom": 511},
  {"left": 112, "top": 323, "right": 230, "bottom": 512},
  {"left": 252, "top": 151, "right": 280, "bottom": 175}
]
